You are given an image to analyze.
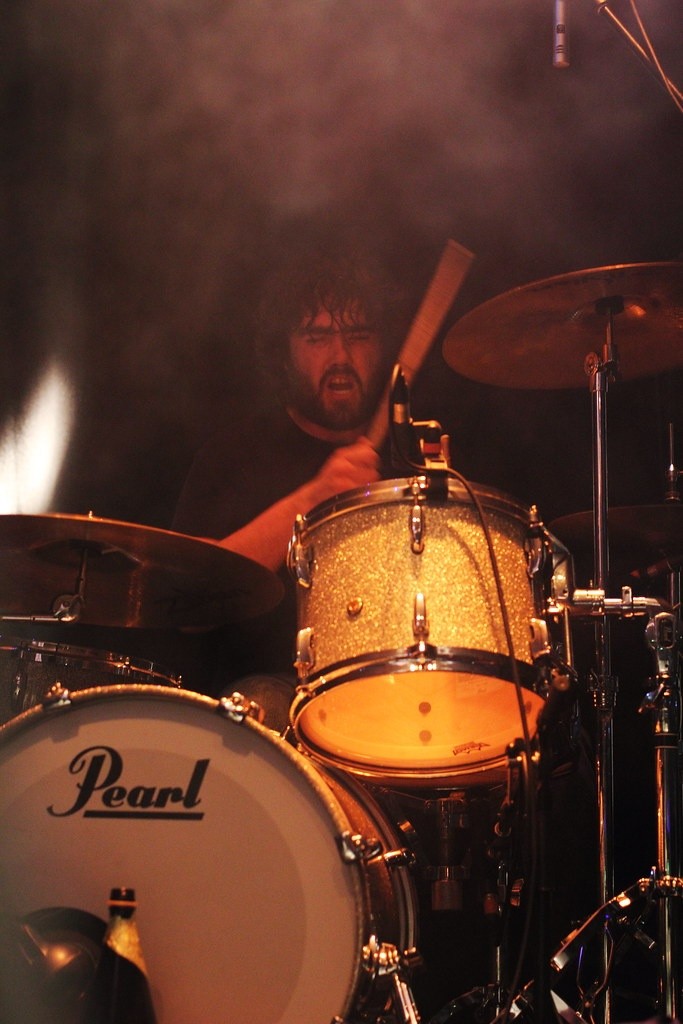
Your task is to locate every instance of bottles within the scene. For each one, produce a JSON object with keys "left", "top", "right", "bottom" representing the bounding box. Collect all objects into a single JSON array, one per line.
[{"left": 85, "top": 885, "right": 158, "bottom": 1024}]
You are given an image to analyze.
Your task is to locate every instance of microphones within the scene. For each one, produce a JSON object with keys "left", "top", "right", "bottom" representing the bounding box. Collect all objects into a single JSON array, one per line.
[
  {"left": 552, "top": 0, "right": 571, "bottom": 68},
  {"left": 389, "top": 372, "right": 423, "bottom": 475}
]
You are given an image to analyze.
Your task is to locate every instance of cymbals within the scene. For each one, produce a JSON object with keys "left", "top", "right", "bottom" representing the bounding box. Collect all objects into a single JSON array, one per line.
[
  {"left": 546, "top": 503, "right": 683, "bottom": 573},
  {"left": 0, "top": 513, "right": 285, "bottom": 632},
  {"left": 441, "top": 260, "right": 683, "bottom": 393}
]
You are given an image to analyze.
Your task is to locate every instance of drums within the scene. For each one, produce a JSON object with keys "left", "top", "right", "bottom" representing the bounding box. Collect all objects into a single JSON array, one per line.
[
  {"left": 288, "top": 474, "right": 575, "bottom": 791},
  {"left": 0, "top": 679, "right": 420, "bottom": 1024},
  {"left": 8, "top": 637, "right": 181, "bottom": 716}
]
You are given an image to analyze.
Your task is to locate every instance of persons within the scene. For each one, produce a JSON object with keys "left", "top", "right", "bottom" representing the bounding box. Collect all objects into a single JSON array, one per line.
[{"left": 169, "top": 281, "right": 455, "bottom": 738}]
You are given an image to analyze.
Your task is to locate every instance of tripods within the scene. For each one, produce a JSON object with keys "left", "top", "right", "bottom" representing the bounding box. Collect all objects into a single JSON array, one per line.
[{"left": 427, "top": 301, "right": 683, "bottom": 1024}]
